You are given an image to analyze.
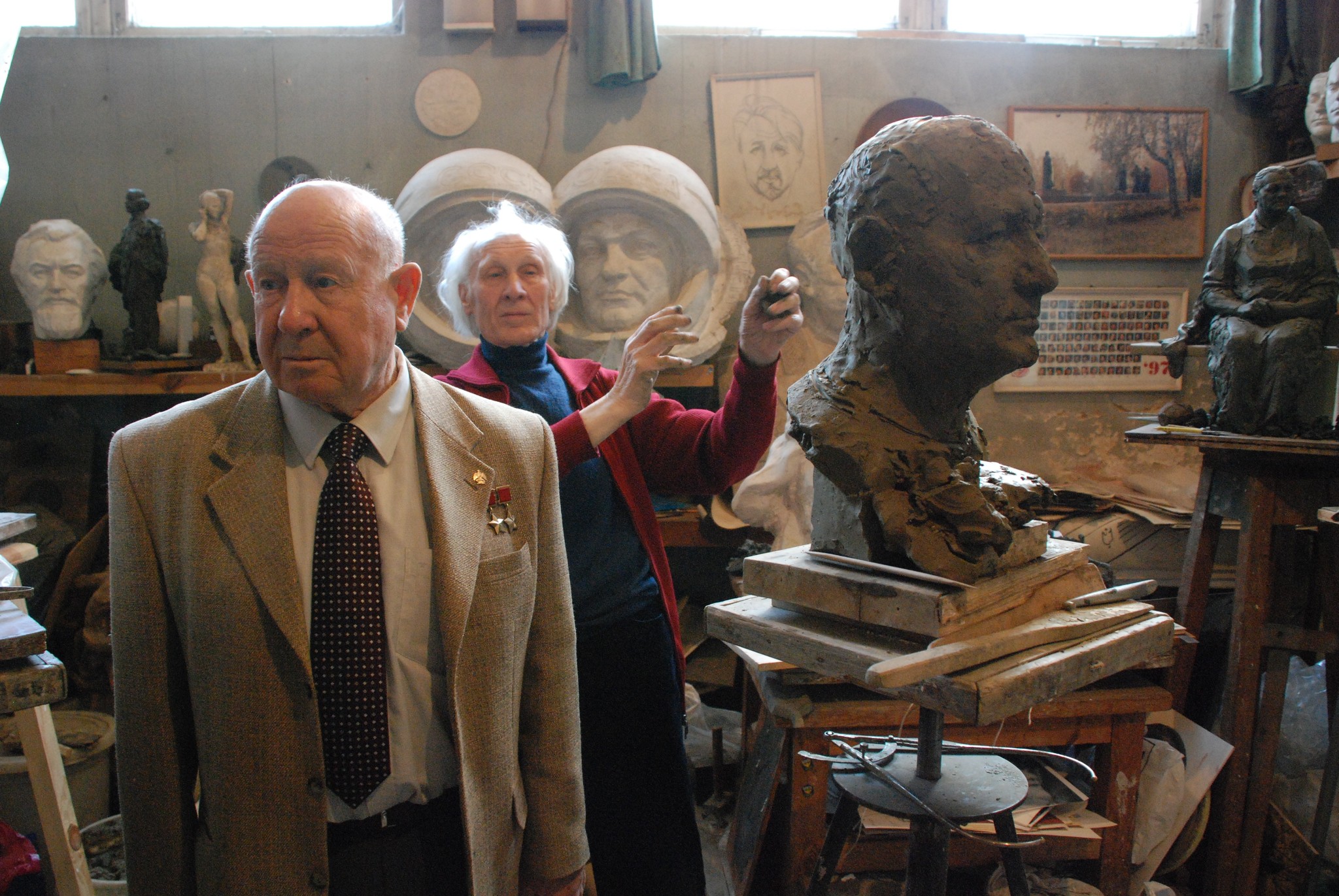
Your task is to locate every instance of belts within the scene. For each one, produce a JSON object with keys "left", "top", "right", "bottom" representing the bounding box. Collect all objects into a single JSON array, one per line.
[{"left": 326, "top": 786, "right": 461, "bottom": 846}]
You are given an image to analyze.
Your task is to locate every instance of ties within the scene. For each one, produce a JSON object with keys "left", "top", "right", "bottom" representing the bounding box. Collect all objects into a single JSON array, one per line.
[{"left": 310, "top": 422, "right": 391, "bottom": 805}]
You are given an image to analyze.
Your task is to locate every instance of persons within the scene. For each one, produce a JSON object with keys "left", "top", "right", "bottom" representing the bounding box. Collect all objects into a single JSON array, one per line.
[
  {"left": 784, "top": 114, "right": 1055, "bottom": 582},
  {"left": 109, "top": 180, "right": 595, "bottom": 896},
  {"left": 1033, "top": 300, "right": 1169, "bottom": 375},
  {"left": 110, "top": 188, "right": 169, "bottom": 362},
  {"left": 392, "top": 146, "right": 848, "bottom": 497},
  {"left": 431, "top": 201, "right": 805, "bottom": 896},
  {"left": 1201, "top": 58, "right": 1339, "bottom": 438},
  {"left": 187, "top": 188, "right": 257, "bottom": 372},
  {"left": 10, "top": 219, "right": 108, "bottom": 339}
]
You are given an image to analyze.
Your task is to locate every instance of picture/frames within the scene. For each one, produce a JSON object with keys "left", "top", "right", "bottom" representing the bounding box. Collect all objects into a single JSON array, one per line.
[
  {"left": 1006, "top": 104, "right": 1205, "bottom": 260},
  {"left": 711, "top": 70, "right": 828, "bottom": 230}
]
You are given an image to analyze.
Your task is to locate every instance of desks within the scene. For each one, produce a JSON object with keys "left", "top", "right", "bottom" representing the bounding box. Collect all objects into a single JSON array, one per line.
[{"left": 738, "top": 654, "right": 1167, "bottom": 895}]
